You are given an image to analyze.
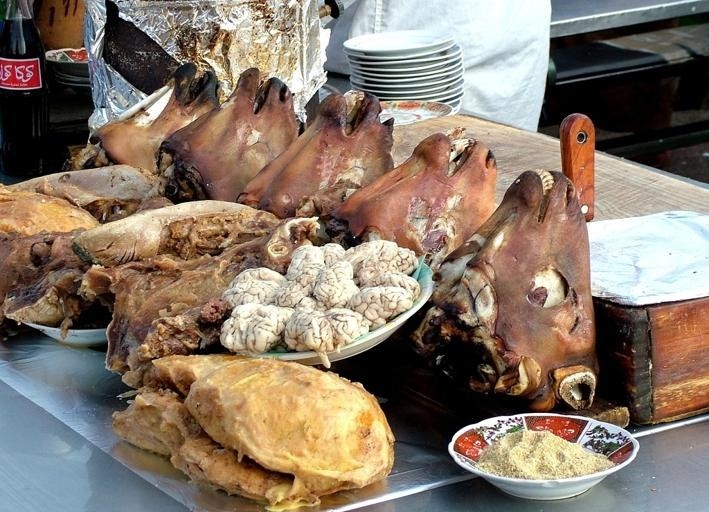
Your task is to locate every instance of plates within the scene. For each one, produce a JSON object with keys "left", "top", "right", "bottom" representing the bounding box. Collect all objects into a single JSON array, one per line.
[
  {"left": 243, "top": 253, "right": 437, "bottom": 366},
  {"left": 19, "top": 312, "right": 127, "bottom": 348},
  {"left": 340, "top": 30, "right": 466, "bottom": 137},
  {"left": 46, "top": 48, "right": 97, "bottom": 91},
  {"left": 448, "top": 412, "right": 640, "bottom": 502}
]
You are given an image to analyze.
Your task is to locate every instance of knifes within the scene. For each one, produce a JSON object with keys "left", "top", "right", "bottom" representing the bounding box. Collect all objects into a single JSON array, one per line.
[{"left": 560, "top": 114, "right": 595, "bottom": 223}]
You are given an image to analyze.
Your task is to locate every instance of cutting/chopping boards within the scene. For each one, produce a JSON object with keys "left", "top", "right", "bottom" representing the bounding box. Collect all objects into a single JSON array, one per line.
[{"left": 389, "top": 111, "right": 709, "bottom": 314}]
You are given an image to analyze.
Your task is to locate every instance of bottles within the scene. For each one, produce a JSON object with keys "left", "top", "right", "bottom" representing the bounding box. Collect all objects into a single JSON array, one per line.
[{"left": 1, "top": 1, "right": 48, "bottom": 183}]
